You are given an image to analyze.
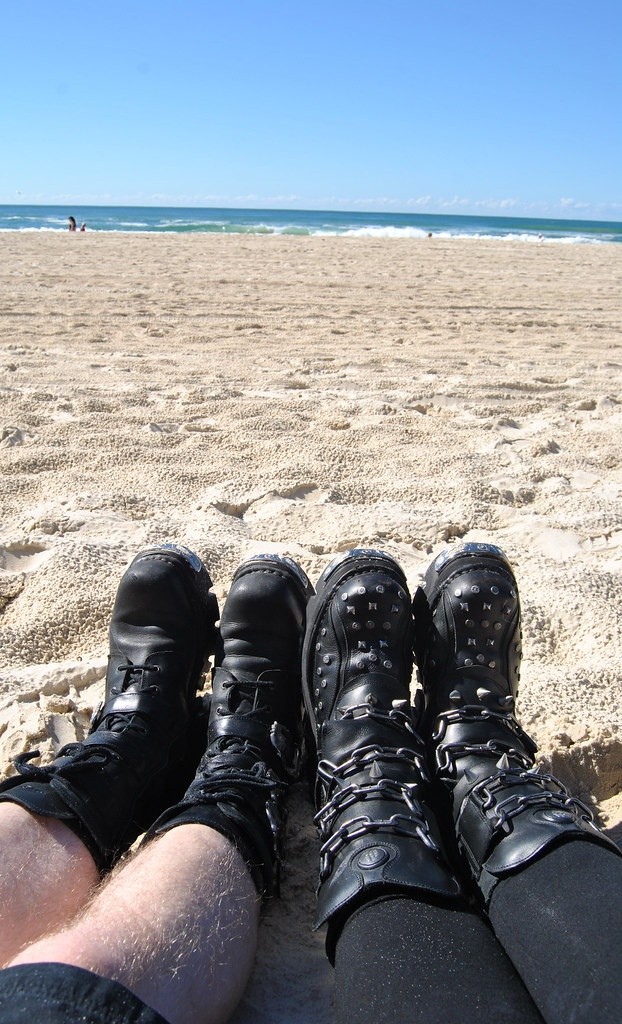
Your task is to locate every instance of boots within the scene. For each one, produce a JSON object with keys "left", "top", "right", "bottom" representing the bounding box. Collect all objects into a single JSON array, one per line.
[
  {"left": 136, "top": 552, "right": 317, "bottom": 925},
  {"left": 0, "top": 546, "right": 220, "bottom": 880},
  {"left": 301, "top": 549, "right": 486, "bottom": 969},
  {"left": 409, "top": 542, "right": 622, "bottom": 903}
]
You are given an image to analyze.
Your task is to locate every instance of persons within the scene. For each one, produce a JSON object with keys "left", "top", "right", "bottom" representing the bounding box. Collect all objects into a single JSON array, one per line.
[
  {"left": 68, "top": 216, "right": 76, "bottom": 231},
  {"left": 0, "top": 543, "right": 314, "bottom": 1024},
  {"left": 80, "top": 223, "right": 86, "bottom": 231},
  {"left": 301, "top": 543, "right": 622, "bottom": 1024}
]
